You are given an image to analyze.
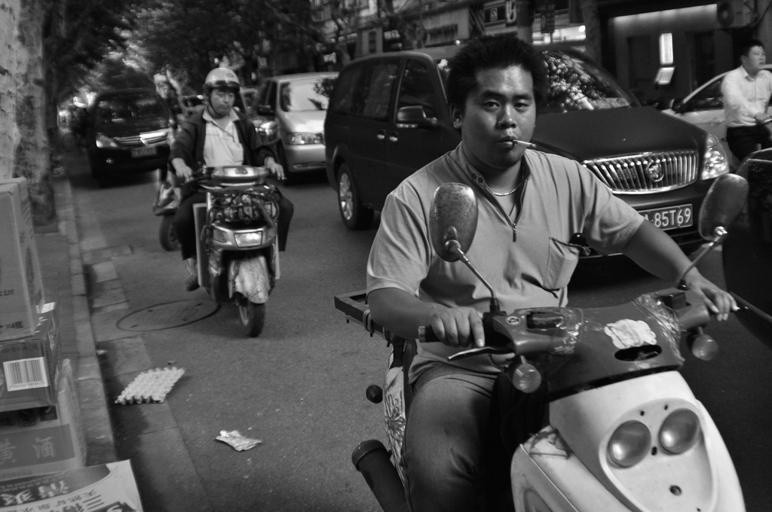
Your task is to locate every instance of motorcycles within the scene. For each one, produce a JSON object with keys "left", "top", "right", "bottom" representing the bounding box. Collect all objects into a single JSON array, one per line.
[
  {"left": 176, "top": 163, "right": 288, "bottom": 339},
  {"left": 331, "top": 171, "right": 751, "bottom": 512}
]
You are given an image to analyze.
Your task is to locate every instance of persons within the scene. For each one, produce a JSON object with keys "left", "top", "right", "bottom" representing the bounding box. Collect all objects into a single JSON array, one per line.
[
  {"left": 720, "top": 40, "right": 772, "bottom": 161},
  {"left": 167, "top": 67, "right": 294, "bottom": 291},
  {"left": 367, "top": 38, "right": 737, "bottom": 512}
]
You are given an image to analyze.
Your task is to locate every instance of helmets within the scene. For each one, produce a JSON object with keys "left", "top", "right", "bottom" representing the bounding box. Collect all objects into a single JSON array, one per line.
[{"left": 205, "top": 67, "right": 240, "bottom": 89}]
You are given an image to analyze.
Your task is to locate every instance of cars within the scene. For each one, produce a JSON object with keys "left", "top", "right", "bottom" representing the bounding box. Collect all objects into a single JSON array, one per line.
[
  {"left": 662, "top": 62, "right": 772, "bottom": 175},
  {"left": 249, "top": 70, "right": 340, "bottom": 180},
  {"left": 85, "top": 86, "right": 180, "bottom": 186}
]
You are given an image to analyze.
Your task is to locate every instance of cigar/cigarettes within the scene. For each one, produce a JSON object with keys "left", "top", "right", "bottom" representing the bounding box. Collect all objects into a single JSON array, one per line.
[{"left": 513, "top": 140, "right": 537, "bottom": 149}]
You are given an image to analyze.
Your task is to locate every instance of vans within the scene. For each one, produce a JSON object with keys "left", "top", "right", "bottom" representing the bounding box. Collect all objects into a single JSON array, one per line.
[{"left": 321, "top": 40, "right": 732, "bottom": 273}]
